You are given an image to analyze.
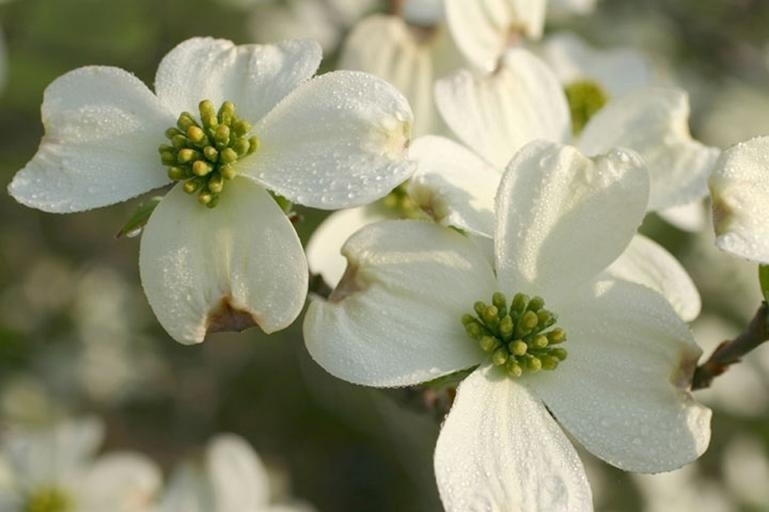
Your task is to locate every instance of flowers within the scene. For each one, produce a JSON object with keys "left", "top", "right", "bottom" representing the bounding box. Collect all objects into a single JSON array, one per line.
[{"left": 0, "top": 2, "right": 769, "bottom": 512}]
